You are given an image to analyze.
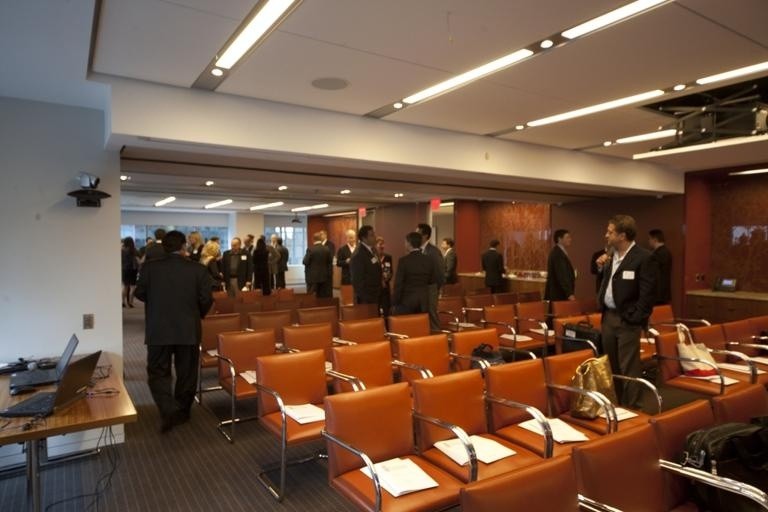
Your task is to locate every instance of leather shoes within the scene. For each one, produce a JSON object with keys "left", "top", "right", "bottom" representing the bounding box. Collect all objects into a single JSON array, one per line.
[{"left": 161, "top": 403, "right": 190, "bottom": 435}]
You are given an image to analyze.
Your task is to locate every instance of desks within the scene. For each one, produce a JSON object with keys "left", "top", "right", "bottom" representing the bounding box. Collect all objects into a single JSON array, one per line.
[{"left": 0, "top": 351, "right": 138, "bottom": 511}]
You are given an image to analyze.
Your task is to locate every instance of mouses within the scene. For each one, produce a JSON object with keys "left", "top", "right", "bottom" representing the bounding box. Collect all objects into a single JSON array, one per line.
[{"left": 9, "top": 385, "right": 34, "bottom": 397}]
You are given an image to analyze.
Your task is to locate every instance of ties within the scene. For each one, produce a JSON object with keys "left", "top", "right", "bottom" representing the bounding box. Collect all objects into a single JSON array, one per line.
[{"left": 371, "top": 248, "right": 376, "bottom": 255}]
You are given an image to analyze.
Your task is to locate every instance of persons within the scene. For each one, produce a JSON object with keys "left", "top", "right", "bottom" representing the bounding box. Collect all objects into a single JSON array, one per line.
[
  {"left": 481, "top": 239, "right": 506, "bottom": 294},
  {"left": 595, "top": 213, "right": 658, "bottom": 409},
  {"left": 121, "top": 228, "right": 164, "bottom": 308},
  {"left": 646, "top": 228, "right": 673, "bottom": 305},
  {"left": 132, "top": 230, "right": 213, "bottom": 433},
  {"left": 544, "top": 228, "right": 576, "bottom": 330},
  {"left": 591, "top": 245, "right": 608, "bottom": 275},
  {"left": 186, "top": 230, "right": 289, "bottom": 298},
  {"left": 301, "top": 221, "right": 458, "bottom": 332}
]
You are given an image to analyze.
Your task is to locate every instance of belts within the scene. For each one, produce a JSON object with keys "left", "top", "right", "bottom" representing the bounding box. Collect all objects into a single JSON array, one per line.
[{"left": 230, "top": 275, "right": 237, "bottom": 278}]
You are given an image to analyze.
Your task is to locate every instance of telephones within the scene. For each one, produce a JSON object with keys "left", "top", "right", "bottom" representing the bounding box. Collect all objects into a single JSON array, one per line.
[{"left": 714, "top": 276, "right": 737, "bottom": 293}]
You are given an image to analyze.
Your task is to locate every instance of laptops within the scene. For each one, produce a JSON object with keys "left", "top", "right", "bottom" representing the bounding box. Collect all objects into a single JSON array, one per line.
[
  {"left": 11, "top": 332, "right": 80, "bottom": 388},
  {"left": 0, "top": 349, "right": 105, "bottom": 417}
]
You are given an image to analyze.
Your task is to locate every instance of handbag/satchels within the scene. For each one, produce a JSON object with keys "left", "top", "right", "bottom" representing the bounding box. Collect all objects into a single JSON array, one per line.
[
  {"left": 641, "top": 356, "right": 660, "bottom": 386},
  {"left": 563, "top": 321, "right": 602, "bottom": 358},
  {"left": 676, "top": 324, "right": 719, "bottom": 376},
  {"left": 569, "top": 353, "right": 619, "bottom": 420},
  {"left": 682, "top": 422, "right": 768, "bottom": 511},
  {"left": 471, "top": 342, "right": 506, "bottom": 368}
]
